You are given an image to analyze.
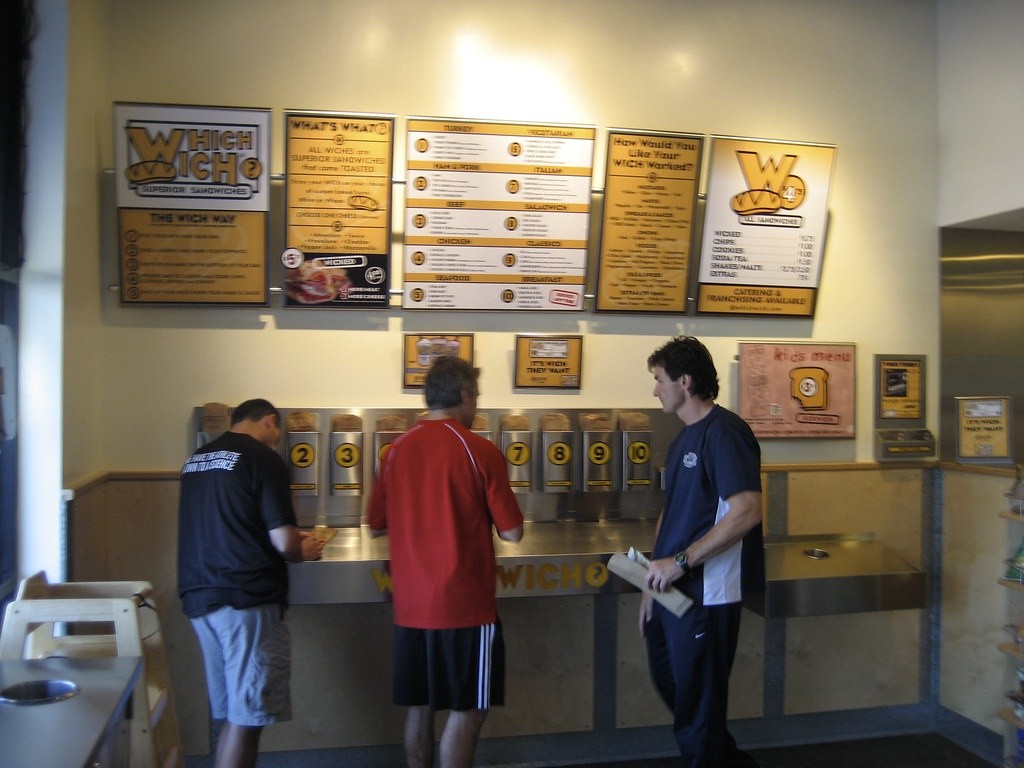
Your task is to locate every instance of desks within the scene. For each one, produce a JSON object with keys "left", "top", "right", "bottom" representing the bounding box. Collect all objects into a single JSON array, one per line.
[{"left": 286, "top": 522, "right": 655, "bottom": 605}]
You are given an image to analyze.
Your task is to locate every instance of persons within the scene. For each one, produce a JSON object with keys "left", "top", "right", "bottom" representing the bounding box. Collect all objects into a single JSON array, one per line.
[
  {"left": 177, "top": 398, "right": 325, "bottom": 768},
  {"left": 638, "top": 334, "right": 767, "bottom": 768},
  {"left": 365, "top": 355, "right": 525, "bottom": 768}
]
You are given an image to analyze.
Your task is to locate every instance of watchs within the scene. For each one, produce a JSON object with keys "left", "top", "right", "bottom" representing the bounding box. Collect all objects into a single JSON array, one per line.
[{"left": 674, "top": 553, "right": 691, "bottom": 572}]
